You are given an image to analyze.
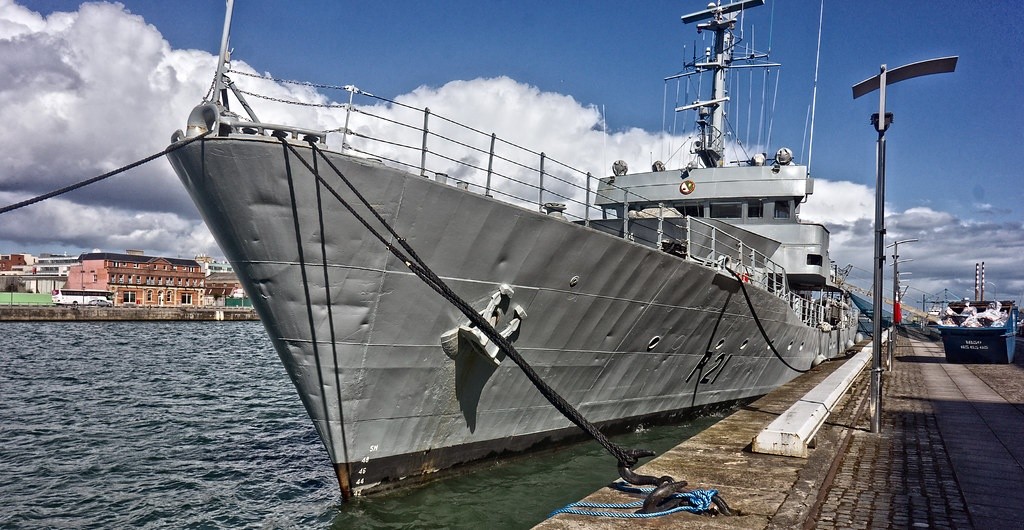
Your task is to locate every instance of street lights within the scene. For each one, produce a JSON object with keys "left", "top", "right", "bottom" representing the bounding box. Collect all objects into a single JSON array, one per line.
[
  {"left": 889, "top": 259, "right": 915, "bottom": 343},
  {"left": 851, "top": 56, "right": 959, "bottom": 434},
  {"left": 886, "top": 238, "right": 919, "bottom": 350}
]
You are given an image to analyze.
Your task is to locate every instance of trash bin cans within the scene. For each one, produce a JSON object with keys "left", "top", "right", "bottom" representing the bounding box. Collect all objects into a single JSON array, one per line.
[{"left": 938, "top": 301, "right": 1017, "bottom": 365}]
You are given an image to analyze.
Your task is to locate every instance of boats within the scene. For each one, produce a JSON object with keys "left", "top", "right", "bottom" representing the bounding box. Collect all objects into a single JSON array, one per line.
[{"left": 163, "top": 0, "right": 862, "bottom": 502}]
